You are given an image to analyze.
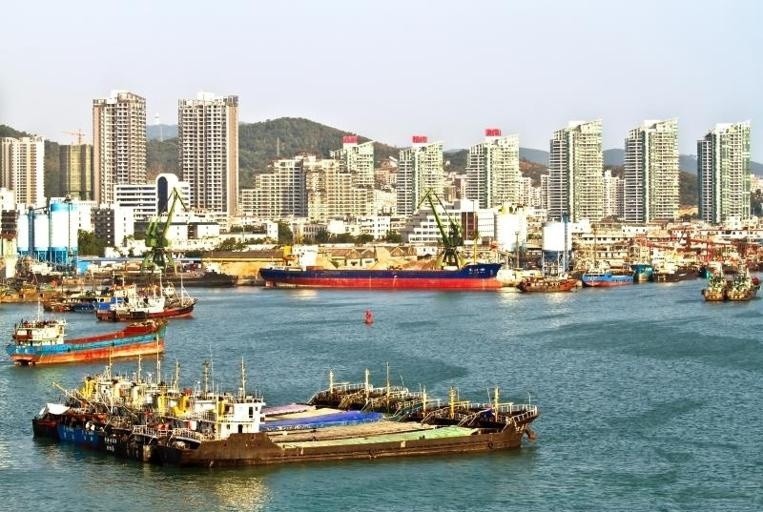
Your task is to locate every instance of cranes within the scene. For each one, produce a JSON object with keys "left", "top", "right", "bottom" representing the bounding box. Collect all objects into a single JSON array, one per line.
[{"left": 68, "top": 129, "right": 86, "bottom": 145}]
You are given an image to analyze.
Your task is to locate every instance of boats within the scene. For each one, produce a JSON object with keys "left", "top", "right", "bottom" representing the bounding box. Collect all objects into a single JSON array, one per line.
[
  {"left": 0, "top": 187, "right": 238, "bottom": 320},
  {"left": 520, "top": 262, "right": 698, "bottom": 291},
  {"left": 6, "top": 315, "right": 168, "bottom": 366},
  {"left": 701, "top": 256, "right": 760, "bottom": 301},
  {"left": 259, "top": 187, "right": 503, "bottom": 290}
]
